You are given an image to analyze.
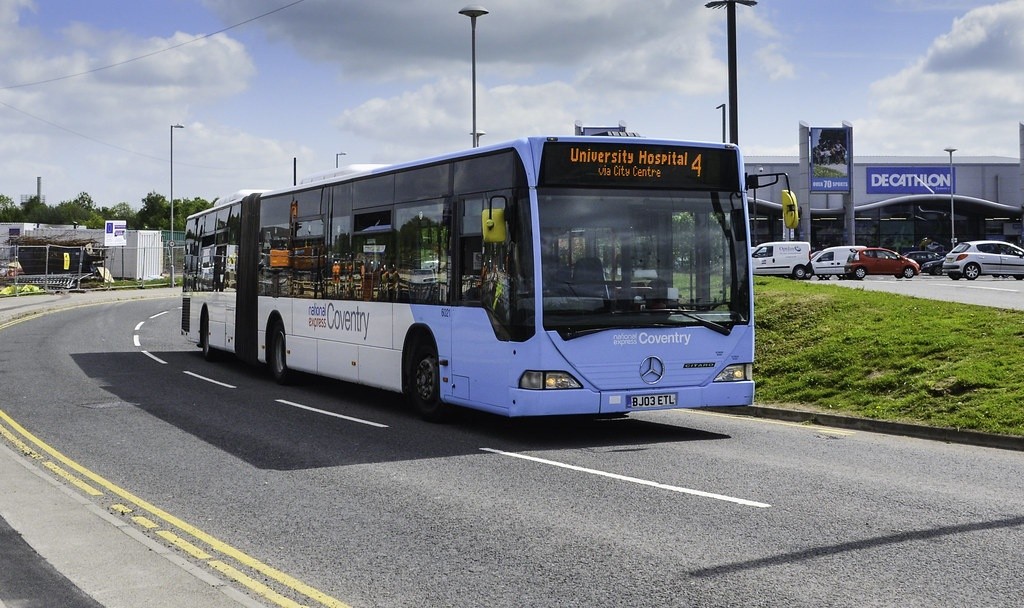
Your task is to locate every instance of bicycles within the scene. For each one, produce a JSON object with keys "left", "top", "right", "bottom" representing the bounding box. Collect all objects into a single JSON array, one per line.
[{"left": 815, "top": 149, "right": 846, "bottom": 165}]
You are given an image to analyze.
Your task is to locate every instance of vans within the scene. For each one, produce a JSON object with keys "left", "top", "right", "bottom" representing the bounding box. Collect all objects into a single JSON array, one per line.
[
  {"left": 751, "top": 241, "right": 813, "bottom": 280},
  {"left": 808, "top": 245, "right": 868, "bottom": 279}
]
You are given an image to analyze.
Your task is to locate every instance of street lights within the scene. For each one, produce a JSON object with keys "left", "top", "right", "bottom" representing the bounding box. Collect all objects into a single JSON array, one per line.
[
  {"left": 458, "top": 5, "right": 489, "bottom": 146},
  {"left": 169, "top": 123, "right": 187, "bottom": 286},
  {"left": 944, "top": 146, "right": 958, "bottom": 247},
  {"left": 334, "top": 150, "right": 347, "bottom": 171}
]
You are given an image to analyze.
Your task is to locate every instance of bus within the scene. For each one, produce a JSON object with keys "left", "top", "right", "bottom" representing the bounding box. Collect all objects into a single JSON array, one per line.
[{"left": 180, "top": 134, "right": 800, "bottom": 423}]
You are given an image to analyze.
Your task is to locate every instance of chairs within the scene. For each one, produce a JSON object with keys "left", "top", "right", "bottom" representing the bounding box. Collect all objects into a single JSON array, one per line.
[{"left": 574, "top": 254, "right": 609, "bottom": 299}]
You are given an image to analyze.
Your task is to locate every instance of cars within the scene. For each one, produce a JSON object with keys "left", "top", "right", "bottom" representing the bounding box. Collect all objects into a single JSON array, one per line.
[
  {"left": 843, "top": 247, "right": 920, "bottom": 280},
  {"left": 903, "top": 250, "right": 942, "bottom": 267},
  {"left": 920, "top": 256, "right": 945, "bottom": 276},
  {"left": 941, "top": 238, "right": 1024, "bottom": 281}
]
